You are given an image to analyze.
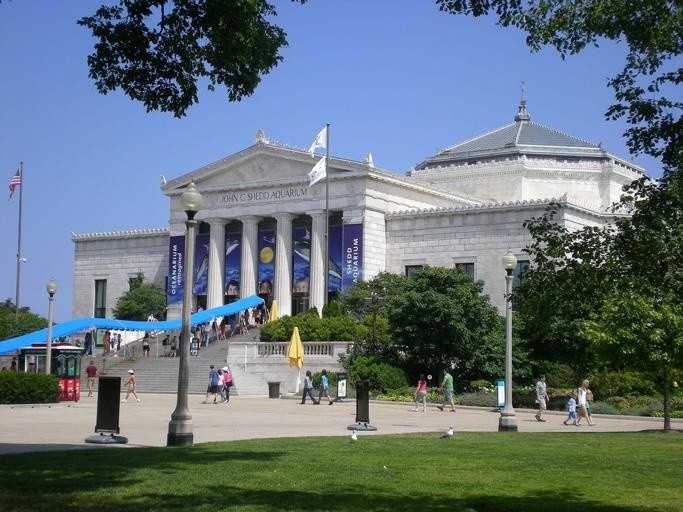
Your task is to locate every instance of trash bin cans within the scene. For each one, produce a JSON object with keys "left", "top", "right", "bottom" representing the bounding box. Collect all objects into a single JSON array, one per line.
[{"left": 268, "top": 382, "right": 279, "bottom": 398}]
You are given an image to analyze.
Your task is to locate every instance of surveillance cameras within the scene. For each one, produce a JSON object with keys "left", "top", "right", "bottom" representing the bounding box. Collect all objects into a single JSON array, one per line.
[{"left": 20, "top": 257, "right": 27, "bottom": 263}]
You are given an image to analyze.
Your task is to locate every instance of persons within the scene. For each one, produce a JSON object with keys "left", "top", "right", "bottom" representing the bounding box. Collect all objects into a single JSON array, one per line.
[
  {"left": 294, "top": 278, "right": 307, "bottom": 293},
  {"left": 85, "top": 305, "right": 270, "bottom": 358},
  {"left": 414, "top": 367, "right": 456, "bottom": 412},
  {"left": 200, "top": 364, "right": 233, "bottom": 404},
  {"left": 121, "top": 369, "right": 141, "bottom": 403},
  {"left": 535, "top": 374, "right": 594, "bottom": 426},
  {"left": 258, "top": 279, "right": 271, "bottom": 295},
  {"left": 301, "top": 368, "right": 333, "bottom": 405},
  {"left": 86, "top": 360, "right": 98, "bottom": 397},
  {"left": 223, "top": 280, "right": 238, "bottom": 294}
]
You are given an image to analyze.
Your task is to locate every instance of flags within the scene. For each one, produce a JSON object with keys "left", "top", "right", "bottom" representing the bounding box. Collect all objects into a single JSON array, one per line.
[
  {"left": 308, "top": 123, "right": 327, "bottom": 159},
  {"left": 8, "top": 161, "right": 21, "bottom": 200},
  {"left": 307, "top": 155, "right": 326, "bottom": 187}
]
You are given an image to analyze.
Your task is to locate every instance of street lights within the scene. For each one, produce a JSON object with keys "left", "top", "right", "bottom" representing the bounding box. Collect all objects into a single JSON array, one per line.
[
  {"left": 497, "top": 250, "right": 518, "bottom": 434},
  {"left": 165, "top": 182, "right": 203, "bottom": 446},
  {"left": 46, "top": 275, "right": 57, "bottom": 375}
]
